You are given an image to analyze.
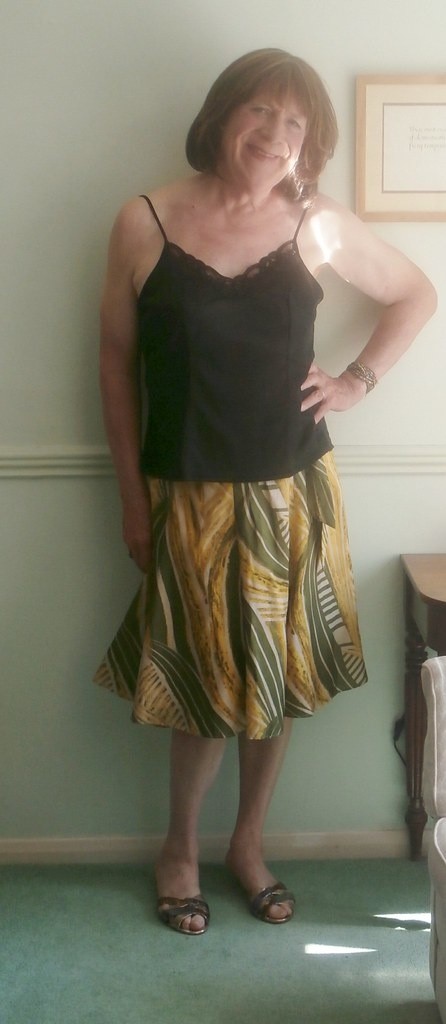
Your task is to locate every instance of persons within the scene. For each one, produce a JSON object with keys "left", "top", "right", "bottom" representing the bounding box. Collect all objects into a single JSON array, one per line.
[{"left": 94, "top": 46, "right": 440, "bottom": 937}]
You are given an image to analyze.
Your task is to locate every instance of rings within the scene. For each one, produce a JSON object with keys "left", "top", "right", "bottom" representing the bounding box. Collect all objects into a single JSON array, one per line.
[{"left": 320, "top": 390, "right": 326, "bottom": 399}]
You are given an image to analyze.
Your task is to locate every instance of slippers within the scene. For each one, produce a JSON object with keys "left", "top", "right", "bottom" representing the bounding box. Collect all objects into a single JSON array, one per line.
[
  {"left": 156, "top": 895, "right": 210, "bottom": 934},
  {"left": 251, "top": 881, "right": 295, "bottom": 923}
]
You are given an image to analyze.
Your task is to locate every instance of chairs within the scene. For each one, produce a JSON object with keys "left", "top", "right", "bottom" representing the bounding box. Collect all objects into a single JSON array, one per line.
[{"left": 421, "top": 656, "right": 446, "bottom": 1024}]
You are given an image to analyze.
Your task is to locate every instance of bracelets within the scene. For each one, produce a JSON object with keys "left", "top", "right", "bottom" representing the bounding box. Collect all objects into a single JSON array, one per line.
[{"left": 348, "top": 360, "right": 378, "bottom": 394}]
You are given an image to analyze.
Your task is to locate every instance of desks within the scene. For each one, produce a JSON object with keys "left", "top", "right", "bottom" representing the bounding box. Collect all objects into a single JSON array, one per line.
[{"left": 393, "top": 552, "right": 446, "bottom": 860}]
